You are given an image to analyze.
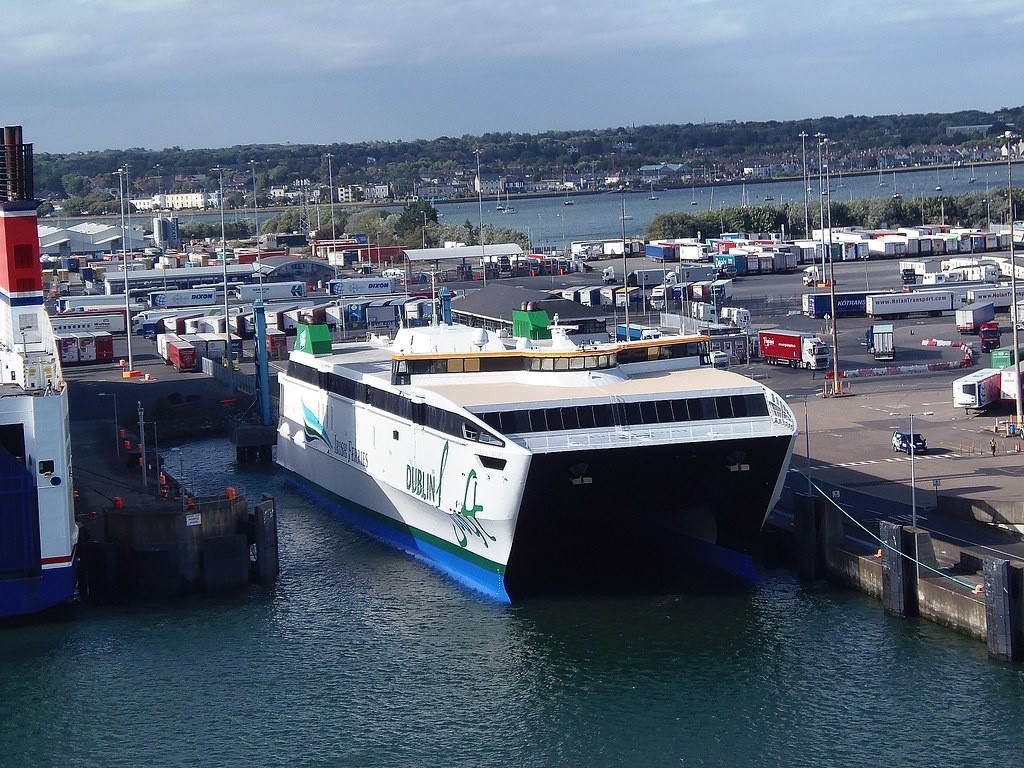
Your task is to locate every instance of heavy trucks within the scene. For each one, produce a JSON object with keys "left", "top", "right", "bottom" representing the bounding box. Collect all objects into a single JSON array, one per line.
[{"left": 758, "top": 329, "right": 830, "bottom": 370}]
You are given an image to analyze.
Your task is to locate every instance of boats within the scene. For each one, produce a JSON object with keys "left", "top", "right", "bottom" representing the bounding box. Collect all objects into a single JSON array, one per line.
[{"left": 271, "top": 265, "right": 798, "bottom": 605}]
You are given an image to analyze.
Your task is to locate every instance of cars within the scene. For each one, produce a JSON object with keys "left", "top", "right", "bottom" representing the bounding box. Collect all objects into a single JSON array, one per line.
[{"left": 891, "top": 431, "right": 927, "bottom": 455}]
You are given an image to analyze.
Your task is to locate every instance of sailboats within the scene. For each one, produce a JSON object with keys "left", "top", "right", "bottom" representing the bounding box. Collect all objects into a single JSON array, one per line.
[
  {"left": 837, "top": 172, "right": 845, "bottom": 188},
  {"left": 807, "top": 171, "right": 814, "bottom": 192},
  {"left": 878, "top": 162, "right": 977, "bottom": 202},
  {"left": 497, "top": 184, "right": 775, "bottom": 220}
]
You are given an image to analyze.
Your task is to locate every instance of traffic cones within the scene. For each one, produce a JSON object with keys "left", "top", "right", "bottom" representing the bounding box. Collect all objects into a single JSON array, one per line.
[{"left": 994, "top": 414, "right": 1021, "bottom": 452}]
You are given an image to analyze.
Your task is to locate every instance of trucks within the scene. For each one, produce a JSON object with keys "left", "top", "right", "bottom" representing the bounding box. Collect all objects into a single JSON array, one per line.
[
  {"left": 979, "top": 322, "right": 1002, "bottom": 353},
  {"left": 55, "top": 277, "right": 396, "bottom": 335},
  {"left": 650, "top": 285, "right": 673, "bottom": 311},
  {"left": 616, "top": 323, "right": 662, "bottom": 342},
  {"left": 802, "top": 266, "right": 836, "bottom": 287},
  {"left": 626, "top": 270, "right": 671, "bottom": 287},
  {"left": 865, "top": 325, "right": 895, "bottom": 359},
  {"left": 709, "top": 280, "right": 733, "bottom": 307}
]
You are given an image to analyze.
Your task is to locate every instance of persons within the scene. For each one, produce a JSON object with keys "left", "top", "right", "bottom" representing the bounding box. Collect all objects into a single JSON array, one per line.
[
  {"left": 813, "top": 372, "right": 815, "bottom": 379},
  {"left": 990, "top": 438, "right": 995, "bottom": 457}
]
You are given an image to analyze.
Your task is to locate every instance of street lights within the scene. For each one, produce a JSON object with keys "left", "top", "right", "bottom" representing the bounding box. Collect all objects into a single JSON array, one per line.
[
  {"left": 423, "top": 226, "right": 428, "bottom": 249},
  {"left": 909, "top": 412, "right": 934, "bottom": 527},
  {"left": 98, "top": 393, "right": 119, "bottom": 456},
  {"left": 421, "top": 211, "right": 426, "bottom": 225},
  {"left": 619, "top": 185, "right": 630, "bottom": 342},
  {"left": 209, "top": 165, "right": 233, "bottom": 369},
  {"left": 798, "top": 130, "right": 839, "bottom": 394},
  {"left": 706, "top": 273, "right": 720, "bottom": 324},
  {"left": 861, "top": 256, "right": 870, "bottom": 291},
  {"left": 326, "top": 153, "right": 337, "bottom": 279},
  {"left": 997, "top": 131, "right": 1024, "bottom": 430},
  {"left": 377, "top": 231, "right": 381, "bottom": 268},
  {"left": 112, "top": 168, "right": 133, "bottom": 370},
  {"left": 473, "top": 149, "right": 486, "bottom": 288},
  {"left": 557, "top": 214, "right": 564, "bottom": 239},
  {"left": 786, "top": 395, "right": 812, "bottom": 494},
  {"left": 137, "top": 421, "right": 160, "bottom": 496},
  {"left": 248, "top": 160, "right": 263, "bottom": 302}
]
